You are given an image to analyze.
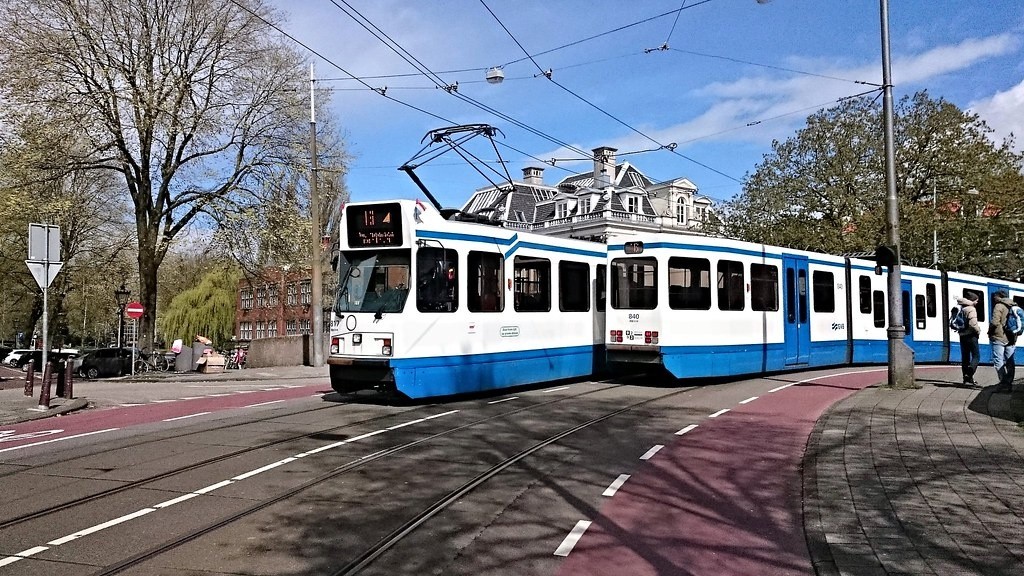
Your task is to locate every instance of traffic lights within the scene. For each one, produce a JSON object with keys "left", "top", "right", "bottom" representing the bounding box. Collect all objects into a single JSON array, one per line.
[{"left": 874, "top": 243, "right": 902, "bottom": 270}]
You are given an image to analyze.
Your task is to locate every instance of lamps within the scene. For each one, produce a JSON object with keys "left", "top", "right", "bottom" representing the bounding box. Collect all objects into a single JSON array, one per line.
[{"left": 486, "top": 67, "right": 505, "bottom": 83}]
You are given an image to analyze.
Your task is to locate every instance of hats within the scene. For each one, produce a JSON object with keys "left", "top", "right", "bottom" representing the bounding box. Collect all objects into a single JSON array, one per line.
[{"left": 967, "top": 292, "right": 978, "bottom": 300}]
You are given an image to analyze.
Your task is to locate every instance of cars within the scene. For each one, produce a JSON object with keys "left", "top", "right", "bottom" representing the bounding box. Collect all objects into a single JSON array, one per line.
[{"left": 2, "top": 349, "right": 136, "bottom": 379}]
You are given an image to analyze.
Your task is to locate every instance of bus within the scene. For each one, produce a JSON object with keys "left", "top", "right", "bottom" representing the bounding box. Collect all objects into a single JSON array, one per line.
[
  {"left": 605, "top": 234, "right": 1024, "bottom": 382},
  {"left": 327, "top": 120, "right": 606, "bottom": 406}
]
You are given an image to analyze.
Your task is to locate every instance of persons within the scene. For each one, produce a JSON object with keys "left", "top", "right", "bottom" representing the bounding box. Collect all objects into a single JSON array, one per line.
[
  {"left": 956, "top": 291, "right": 981, "bottom": 387},
  {"left": 987, "top": 291, "right": 1018, "bottom": 392}
]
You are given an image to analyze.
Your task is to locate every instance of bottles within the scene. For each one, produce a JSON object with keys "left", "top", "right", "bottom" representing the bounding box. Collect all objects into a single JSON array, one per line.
[{"left": 436, "top": 302, "right": 446, "bottom": 311}]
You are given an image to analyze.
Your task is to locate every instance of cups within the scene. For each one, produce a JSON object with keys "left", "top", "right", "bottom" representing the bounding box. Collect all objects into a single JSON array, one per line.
[
  {"left": 376, "top": 284, "right": 384, "bottom": 298},
  {"left": 447, "top": 302, "right": 451, "bottom": 311}
]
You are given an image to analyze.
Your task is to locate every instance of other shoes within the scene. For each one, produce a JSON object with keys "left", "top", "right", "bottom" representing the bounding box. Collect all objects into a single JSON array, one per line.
[
  {"left": 963, "top": 377, "right": 978, "bottom": 386},
  {"left": 1008, "top": 383, "right": 1013, "bottom": 392},
  {"left": 989, "top": 383, "right": 1008, "bottom": 393}
]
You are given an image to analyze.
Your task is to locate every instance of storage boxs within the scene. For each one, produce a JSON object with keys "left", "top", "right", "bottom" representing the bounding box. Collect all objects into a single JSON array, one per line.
[{"left": 195, "top": 358, "right": 225, "bottom": 374}]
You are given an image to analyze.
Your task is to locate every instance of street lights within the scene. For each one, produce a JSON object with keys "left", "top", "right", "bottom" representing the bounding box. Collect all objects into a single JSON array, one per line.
[
  {"left": 58, "top": 310, "right": 67, "bottom": 353},
  {"left": 113, "top": 285, "right": 131, "bottom": 376},
  {"left": 12, "top": 317, "right": 21, "bottom": 348}
]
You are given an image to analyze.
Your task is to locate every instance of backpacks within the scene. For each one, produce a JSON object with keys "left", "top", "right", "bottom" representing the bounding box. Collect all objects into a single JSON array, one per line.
[
  {"left": 949, "top": 305, "right": 970, "bottom": 331},
  {"left": 996, "top": 302, "right": 1024, "bottom": 335}
]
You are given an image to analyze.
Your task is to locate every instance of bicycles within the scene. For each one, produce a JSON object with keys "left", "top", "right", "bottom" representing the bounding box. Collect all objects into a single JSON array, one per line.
[
  {"left": 221, "top": 350, "right": 248, "bottom": 369},
  {"left": 134, "top": 346, "right": 171, "bottom": 375}
]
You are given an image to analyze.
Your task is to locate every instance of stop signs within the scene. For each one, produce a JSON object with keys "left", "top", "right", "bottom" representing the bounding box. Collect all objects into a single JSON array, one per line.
[{"left": 127, "top": 303, "right": 145, "bottom": 321}]
